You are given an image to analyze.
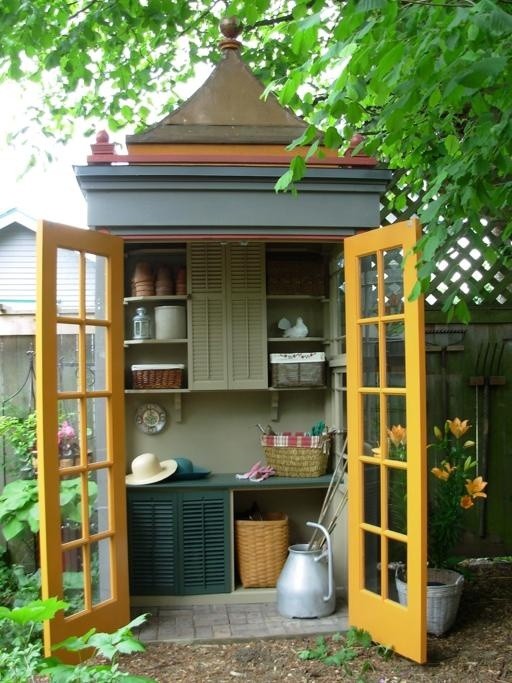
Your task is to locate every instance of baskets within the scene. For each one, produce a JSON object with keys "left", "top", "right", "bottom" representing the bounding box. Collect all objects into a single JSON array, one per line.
[
  {"left": 131, "top": 365, "right": 184, "bottom": 388},
  {"left": 262, "top": 432, "right": 333, "bottom": 477},
  {"left": 237, "top": 512, "right": 289, "bottom": 588}
]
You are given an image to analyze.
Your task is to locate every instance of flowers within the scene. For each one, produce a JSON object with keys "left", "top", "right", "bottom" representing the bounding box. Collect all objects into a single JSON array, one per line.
[{"left": 369, "top": 415, "right": 489, "bottom": 569}]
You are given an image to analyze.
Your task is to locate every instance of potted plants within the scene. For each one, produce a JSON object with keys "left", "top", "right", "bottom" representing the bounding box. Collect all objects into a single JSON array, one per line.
[
  {"left": 0, "top": 479, "right": 97, "bottom": 573},
  {"left": 0, "top": 411, "right": 93, "bottom": 479}
]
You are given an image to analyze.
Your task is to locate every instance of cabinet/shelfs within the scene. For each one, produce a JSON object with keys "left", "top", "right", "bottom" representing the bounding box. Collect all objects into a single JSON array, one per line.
[{"left": 122, "top": 242, "right": 336, "bottom": 606}]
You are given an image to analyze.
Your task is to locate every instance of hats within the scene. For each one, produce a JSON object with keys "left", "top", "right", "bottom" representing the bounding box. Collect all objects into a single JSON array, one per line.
[
  {"left": 125, "top": 453, "right": 178, "bottom": 485},
  {"left": 173, "top": 458, "right": 209, "bottom": 478}
]
